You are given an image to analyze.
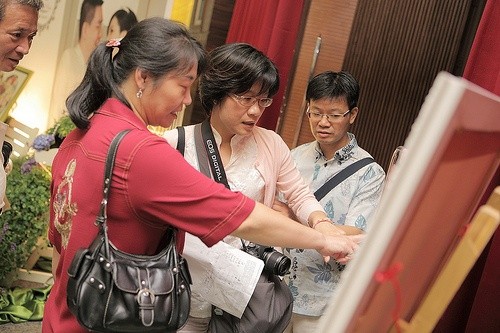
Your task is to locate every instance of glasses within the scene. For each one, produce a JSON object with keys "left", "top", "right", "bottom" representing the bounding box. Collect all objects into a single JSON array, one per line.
[
  {"left": 305, "top": 106, "right": 351, "bottom": 120},
  {"left": 227, "top": 91, "right": 273, "bottom": 107}
]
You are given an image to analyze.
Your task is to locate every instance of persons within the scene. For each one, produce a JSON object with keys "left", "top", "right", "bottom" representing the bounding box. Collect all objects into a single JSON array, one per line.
[
  {"left": 105, "top": 7, "right": 137, "bottom": 45},
  {"left": 40, "top": 19, "right": 363, "bottom": 333},
  {"left": 155, "top": 40, "right": 348, "bottom": 333},
  {"left": 282, "top": 68, "right": 386, "bottom": 333},
  {"left": 49, "top": 0, "right": 105, "bottom": 121},
  {"left": 0, "top": 0, "right": 44, "bottom": 218}
]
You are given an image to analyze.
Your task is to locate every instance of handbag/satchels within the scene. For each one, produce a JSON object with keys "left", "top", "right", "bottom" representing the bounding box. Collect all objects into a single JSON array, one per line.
[
  {"left": 208, "top": 269, "right": 296, "bottom": 333},
  {"left": 66, "top": 129, "right": 192, "bottom": 332}
]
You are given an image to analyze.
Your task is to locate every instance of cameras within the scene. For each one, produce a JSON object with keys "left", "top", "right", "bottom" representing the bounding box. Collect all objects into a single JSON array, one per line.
[{"left": 239, "top": 242, "right": 291, "bottom": 277}]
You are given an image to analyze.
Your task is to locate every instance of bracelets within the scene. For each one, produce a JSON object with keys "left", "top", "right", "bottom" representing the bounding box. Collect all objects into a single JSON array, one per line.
[{"left": 311, "top": 218, "right": 334, "bottom": 229}]
